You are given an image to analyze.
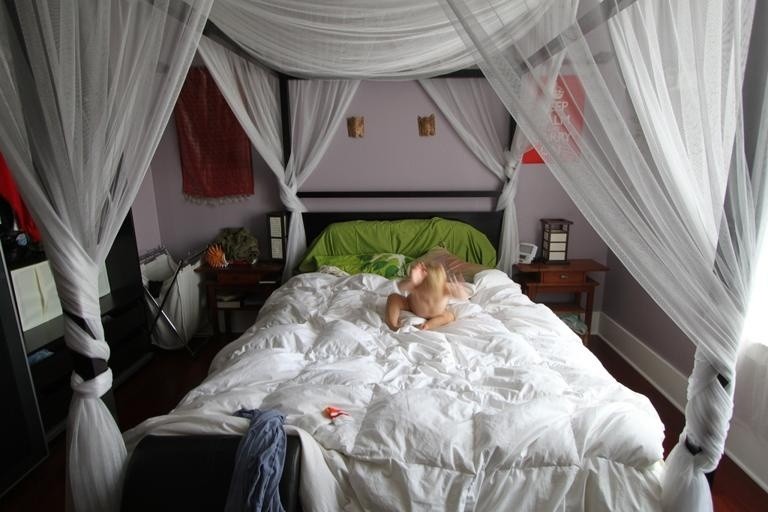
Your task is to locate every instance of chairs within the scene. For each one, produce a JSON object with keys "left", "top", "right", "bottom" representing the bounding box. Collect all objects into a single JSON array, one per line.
[{"left": 122, "top": 434, "right": 302, "bottom": 512}]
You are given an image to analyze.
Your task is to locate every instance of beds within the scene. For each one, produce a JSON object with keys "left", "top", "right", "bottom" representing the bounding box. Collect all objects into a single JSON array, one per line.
[{"left": 0, "top": 1, "right": 768, "bottom": 512}]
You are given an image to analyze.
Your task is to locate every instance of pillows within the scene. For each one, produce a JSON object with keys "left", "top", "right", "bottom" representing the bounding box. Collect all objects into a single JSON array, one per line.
[{"left": 312, "top": 254, "right": 411, "bottom": 279}]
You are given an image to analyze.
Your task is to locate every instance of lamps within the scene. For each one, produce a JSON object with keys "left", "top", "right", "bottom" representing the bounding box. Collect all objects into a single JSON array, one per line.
[
  {"left": 265, "top": 213, "right": 288, "bottom": 263},
  {"left": 540, "top": 218, "right": 573, "bottom": 265}
]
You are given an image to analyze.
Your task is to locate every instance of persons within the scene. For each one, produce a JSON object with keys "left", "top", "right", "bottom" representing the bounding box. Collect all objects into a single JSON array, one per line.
[{"left": 387, "top": 258, "right": 479, "bottom": 330}]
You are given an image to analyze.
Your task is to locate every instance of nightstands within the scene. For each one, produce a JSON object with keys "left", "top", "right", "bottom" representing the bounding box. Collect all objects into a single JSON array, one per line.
[
  {"left": 512, "top": 260, "right": 610, "bottom": 347},
  {"left": 206, "top": 265, "right": 283, "bottom": 345}
]
